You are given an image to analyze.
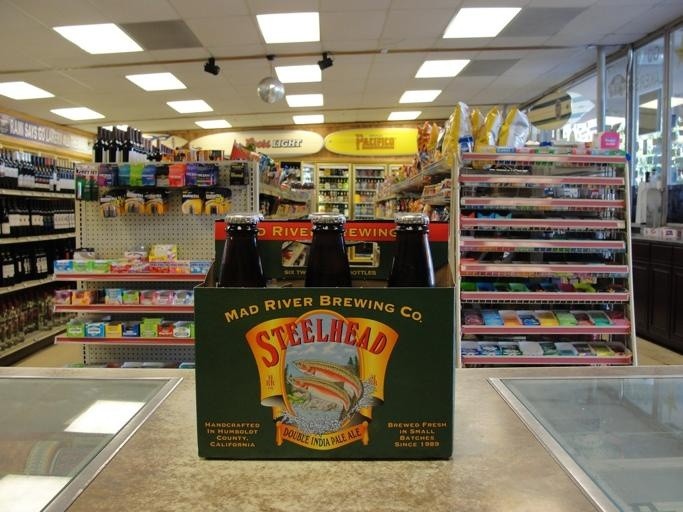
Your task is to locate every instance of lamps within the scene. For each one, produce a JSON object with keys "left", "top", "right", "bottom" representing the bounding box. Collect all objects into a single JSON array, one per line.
[
  {"left": 204, "top": 57, "right": 220, "bottom": 74},
  {"left": 318, "top": 52, "right": 333, "bottom": 70}
]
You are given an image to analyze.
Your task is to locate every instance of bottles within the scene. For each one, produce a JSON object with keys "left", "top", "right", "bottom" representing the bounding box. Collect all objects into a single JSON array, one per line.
[
  {"left": 0, "top": 146, "right": 74, "bottom": 350},
  {"left": 318, "top": 169, "right": 384, "bottom": 216},
  {"left": 389, "top": 213, "right": 435, "bottom": 287},
  {"left": 93, "top": 125, "right": 175, "bottom": 162},
  {"left": 303, "top": 213, "right": 352, "bottom": 287},
  {"left": 218, "top": 214, "right": 266, "bottom": 286}
]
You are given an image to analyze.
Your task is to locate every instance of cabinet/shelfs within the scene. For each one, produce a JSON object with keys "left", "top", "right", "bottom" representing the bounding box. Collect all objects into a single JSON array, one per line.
[
  {"left": 0, "top": 151, "right": 638, "bottom": 369},
  {"left": 632, "top": 239, "right": 683, "bottom": 356}
]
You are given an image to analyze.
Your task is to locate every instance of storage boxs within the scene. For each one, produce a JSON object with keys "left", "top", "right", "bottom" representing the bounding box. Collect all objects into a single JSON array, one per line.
[{"left": 192, "top": 218, "right": 457, "bottom": 461}]
